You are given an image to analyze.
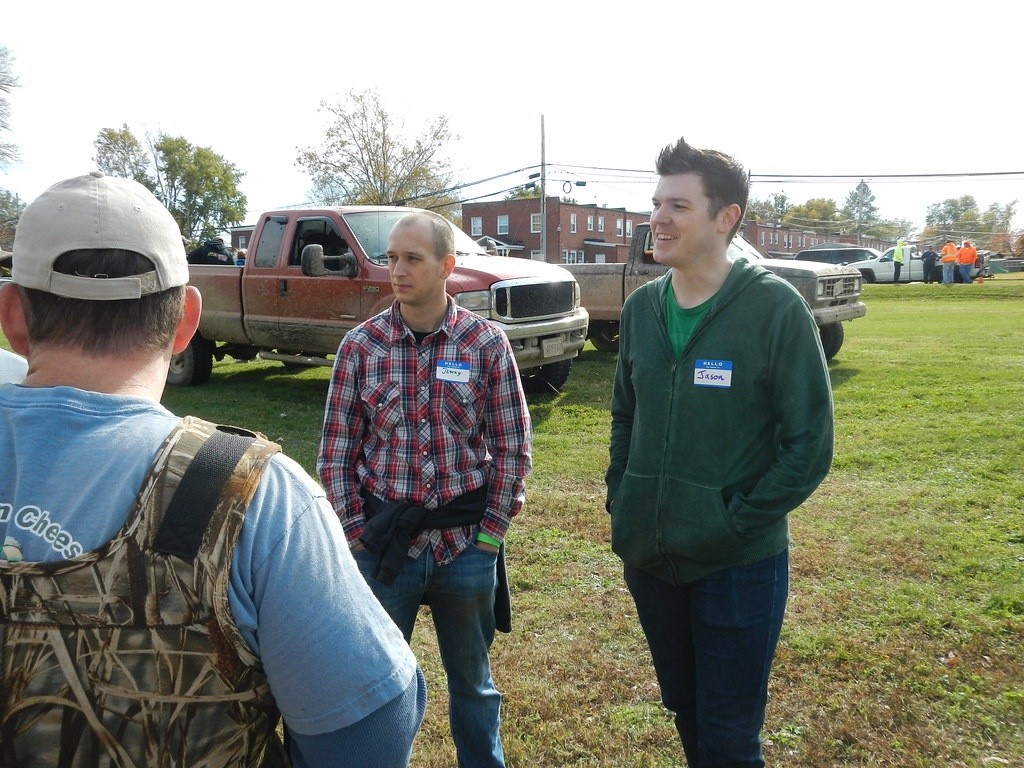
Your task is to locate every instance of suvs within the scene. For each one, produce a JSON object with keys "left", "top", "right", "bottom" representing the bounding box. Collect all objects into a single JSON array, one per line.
[{"left": 792, "top": 247, "right": 881, "bottom": 266}]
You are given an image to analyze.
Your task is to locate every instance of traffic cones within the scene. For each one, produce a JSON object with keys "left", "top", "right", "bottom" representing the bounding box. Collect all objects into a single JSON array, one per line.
[{"left": 978, "top": 276, "right": 983, "bottom": 284}]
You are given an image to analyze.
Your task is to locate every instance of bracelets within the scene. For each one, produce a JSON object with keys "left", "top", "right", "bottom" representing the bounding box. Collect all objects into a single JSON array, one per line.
[{"left": 477, "top": 533, "right": 500, "bottom": 548}]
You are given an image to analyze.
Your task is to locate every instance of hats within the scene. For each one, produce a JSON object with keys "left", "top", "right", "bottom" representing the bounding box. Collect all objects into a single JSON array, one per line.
[{"left": 12, "top": 172, "right": 189, "bottom": 301}]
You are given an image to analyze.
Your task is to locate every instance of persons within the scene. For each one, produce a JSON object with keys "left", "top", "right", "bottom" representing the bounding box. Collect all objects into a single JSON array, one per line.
[
  {"left": 605, "top": 138, "right": 834, "bottom": 768},
  {"left": 234, "top": 248, "right": 260, "bottom": 363},
  {"left": 940, "top": 240, "right": 956, "bottom": 284},
  {"left": 0, "top": 171, "right": 424, "bottom": 768},
  {"left": 187, "top": 236, "right": 235, "bottom": 265},
  {"left": 958, "top": 241, "right": 977, "bottom": 285},
  {"left": 894, "top": 239, "right": 903, "bottom": 283},
  {"left": 316, "top": 208, "right": 531, "bottom": 768},
  {"left": 921, "top": 245, "right": 940, "bottom": 284}
]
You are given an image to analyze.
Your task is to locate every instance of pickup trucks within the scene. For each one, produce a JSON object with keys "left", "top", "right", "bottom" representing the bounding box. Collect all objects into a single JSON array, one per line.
[
  {"left": 549, "top": 222, "right": 866, "bottom": 361},
  {"left": 165, "top": 206, "right": 590, "bottom": 395},
  {"left": 845, "top": 244, "right": 991, "bottom": 283}
]
